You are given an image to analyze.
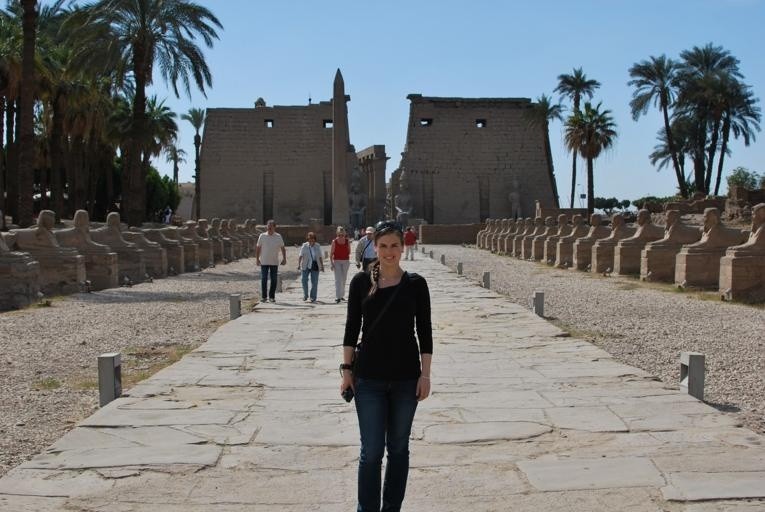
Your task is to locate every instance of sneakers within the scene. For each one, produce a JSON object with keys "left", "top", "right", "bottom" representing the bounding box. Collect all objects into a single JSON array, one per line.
[
  {"left": 261, "top": 297, "right": 267, "bottom": 302},
  {"left": 304, "top": 294, "right": 309, "bottom": 301},
  {"left": 270, "top": 297, "right": 276, "bottom": 303},
  {"left": 310, "top": 298, "right": 315, "bottom": 303},
  {"left": 335, "top": 296, "right": 345, "bottom": 304}
]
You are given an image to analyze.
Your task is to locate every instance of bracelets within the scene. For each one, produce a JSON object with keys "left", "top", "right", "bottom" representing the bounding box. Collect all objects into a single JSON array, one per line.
[
  {"left": 419, "top": 374, "right": 431, "bottom": 380},
  {"left": 339, "top": 362, "right": 353, "bottom": 378}
]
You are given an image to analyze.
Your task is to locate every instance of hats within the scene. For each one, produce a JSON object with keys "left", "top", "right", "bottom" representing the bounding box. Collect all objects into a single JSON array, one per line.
[
  {"left": 311, "top": 260, "right": 319, "bottom": 271},
  {"left": 336, "top": 225, "right": 346, "bottom": 234},
  {"left": 365, "top": 226, "right": 376, "bottom": 234}
]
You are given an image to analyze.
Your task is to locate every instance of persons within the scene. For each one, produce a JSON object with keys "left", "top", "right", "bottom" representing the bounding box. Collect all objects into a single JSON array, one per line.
[
  {"left": 329, "top": 226, "right": 351, "bottom": 304},
  {"left": 255, "top": 219, "right": 287, "bottom": 303},
  {"left": 351, "top": 223, "right": 417, "bottom": 261},
  {"left": 354, "top": 226, "right": 378, "bottom": 273},
  {"left": 339, "top": 218, "right": 434, "bottom": 512},
  {"left": 345, "top": 180, "right": 369, "bottom": 225},
  {"left": 297, "top": 232, "right": 325, "bottom": 303},
  {"left": 393, "top": 180, "right": 416, "bottom": 222}
]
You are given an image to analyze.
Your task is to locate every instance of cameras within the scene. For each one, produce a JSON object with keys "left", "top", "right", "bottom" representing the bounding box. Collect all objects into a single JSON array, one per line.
[{"left": 341, "top": 386, "right": 354, "bottom": 402}]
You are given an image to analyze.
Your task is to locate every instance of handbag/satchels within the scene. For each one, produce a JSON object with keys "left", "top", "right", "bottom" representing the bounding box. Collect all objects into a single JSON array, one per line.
[
  {"left": 350, "top": 346, "right": 363, "bottom": 393},
  {"left": 360, "top": 255, "right": 362, "bottom": 261}
]
